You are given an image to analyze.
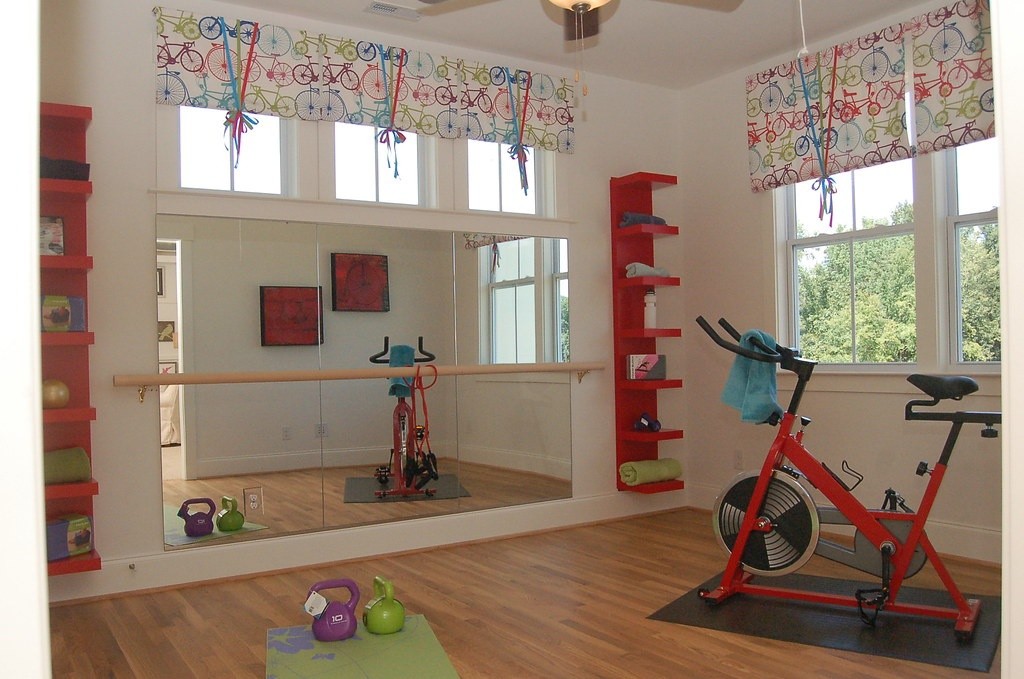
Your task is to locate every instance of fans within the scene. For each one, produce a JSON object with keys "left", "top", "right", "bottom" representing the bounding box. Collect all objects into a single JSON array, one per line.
[{"left": 420, "top": 0, "right": 744, "bottom": 43}]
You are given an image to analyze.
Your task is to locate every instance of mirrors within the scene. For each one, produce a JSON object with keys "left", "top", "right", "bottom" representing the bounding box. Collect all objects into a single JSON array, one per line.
[{"left": 155, "top": 212, "right": 573, "bottom": 552}]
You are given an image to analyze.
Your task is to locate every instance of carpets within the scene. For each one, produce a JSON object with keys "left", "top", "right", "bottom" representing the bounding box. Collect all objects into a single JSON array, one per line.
[
  {"left": 163, "top": 505, "right": 266, "bottom": 546},
  {"left": 646, "top": 569, "right": 1002, "bottom": 674},
  {"left": 266, "top": 614, "right": 459, "bottom": 679},
  {"left": 345, "top": 472, "right": 472, "bottom": 504}
]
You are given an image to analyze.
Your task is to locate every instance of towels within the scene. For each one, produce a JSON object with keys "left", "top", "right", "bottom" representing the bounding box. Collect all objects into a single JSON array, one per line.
[
  {"left": 624, "top": 262, "right": 669, "bottom": 278},
  {"left": 719, "top": 329, "right": 784, "bottom": 425},
  {"left": 619, "top": 212, "right": 666, "bottom": 227},
  {"left": 388, "top": 344, "right": 416, "bottom": 396},
  {"left": 619, "top": 458, "right": 682, "bottom": 486}
]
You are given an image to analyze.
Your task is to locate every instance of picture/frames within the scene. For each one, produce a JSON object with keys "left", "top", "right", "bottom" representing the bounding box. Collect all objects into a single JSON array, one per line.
[
  {"left": 157, "top": 320, "right": 175, "bottom": 342},
  {"left": 158, "top": 359, "right": 180, "bottom": 375},
  {"left": 157, "top": 266, "right": 165, "bottom": 297}
]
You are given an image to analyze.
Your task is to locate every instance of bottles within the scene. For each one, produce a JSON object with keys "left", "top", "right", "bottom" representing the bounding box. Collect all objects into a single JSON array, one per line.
[{"left": 643, "top": 288, "right": 657, "bottom": 329}]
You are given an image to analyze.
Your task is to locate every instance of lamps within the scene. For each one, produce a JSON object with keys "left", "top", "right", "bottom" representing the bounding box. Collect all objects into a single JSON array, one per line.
[{"left": 550, "top": 0, "right": 610, "bottom": 96}]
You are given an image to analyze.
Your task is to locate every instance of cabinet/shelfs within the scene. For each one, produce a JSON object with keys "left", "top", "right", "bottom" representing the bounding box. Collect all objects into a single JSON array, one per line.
[
  {"left": 39, "top": 100, "right": 101, "bottom": 574},
  {"left": 610, "top": 174, "right": 685, "bottom": 493}
]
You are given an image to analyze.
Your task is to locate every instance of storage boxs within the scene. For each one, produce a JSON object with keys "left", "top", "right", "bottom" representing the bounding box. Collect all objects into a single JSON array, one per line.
[
  {"left": 625, "top": 354, "right": 667, "bottom": 380},
  {"left": 40, "top": 295, "right": 85, "bottom": 333}
]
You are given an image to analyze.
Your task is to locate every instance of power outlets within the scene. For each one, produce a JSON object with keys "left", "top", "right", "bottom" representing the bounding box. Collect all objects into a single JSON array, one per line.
[
  {"left": 243, "top": 486, "right": 263, "bottom": 519},
  {"left": 734, "top": 449, "right": 744, "bottom": 470}
]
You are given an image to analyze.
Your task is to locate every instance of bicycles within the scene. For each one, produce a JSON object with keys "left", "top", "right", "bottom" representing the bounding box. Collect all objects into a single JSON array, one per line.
[
  {"left": 696, "top": 315, "right": 1002, "bottom": 640},
  {"left": 369, "top": 336, "right": 440, "bottom": 498}
]
